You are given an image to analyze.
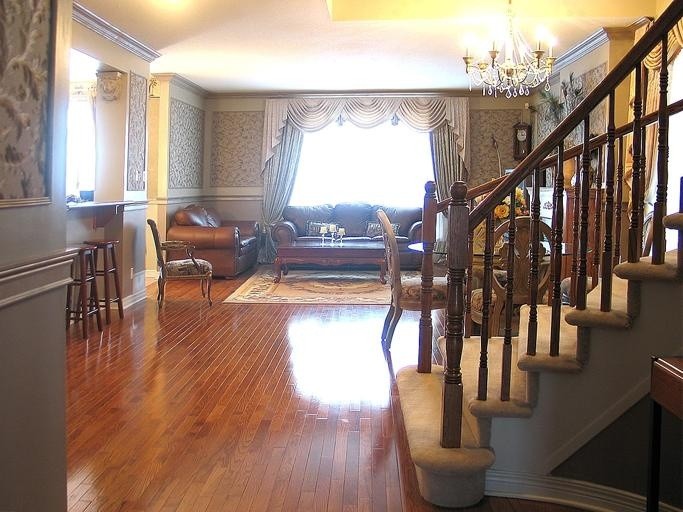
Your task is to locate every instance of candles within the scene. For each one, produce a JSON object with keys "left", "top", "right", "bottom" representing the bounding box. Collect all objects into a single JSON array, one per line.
[
  {"left": 321, "top": 227, "right": 327, "bottom": 232},
  {"left": 339, "top": 227, "right": 345, "bottom": 234},
  {"left": 330, "top": 225, "right": 336, "bottom": 231}
]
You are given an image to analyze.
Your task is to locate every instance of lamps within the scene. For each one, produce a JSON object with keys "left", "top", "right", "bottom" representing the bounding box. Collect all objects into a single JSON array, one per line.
[{"left": 463, "top": 0, "right": 557, "bottom": 97}]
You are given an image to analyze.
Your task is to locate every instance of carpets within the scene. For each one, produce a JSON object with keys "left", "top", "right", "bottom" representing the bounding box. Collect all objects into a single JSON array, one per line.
[{"left": 222, "top": 264, "right": 421, "bottom": 304}]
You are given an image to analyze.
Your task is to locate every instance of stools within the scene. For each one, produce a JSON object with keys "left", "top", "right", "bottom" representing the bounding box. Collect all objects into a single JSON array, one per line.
[
  {"left": 85, "top": 238, "right": 125, "bottom": 324},
  {"left": 66, "top": 244, "right": 103, "bottom": 338}
]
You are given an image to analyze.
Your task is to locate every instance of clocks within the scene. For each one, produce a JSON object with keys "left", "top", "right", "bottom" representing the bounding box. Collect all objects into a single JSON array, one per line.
[{"left": 512, "top": 122, "right": 531, "bottom": 160}]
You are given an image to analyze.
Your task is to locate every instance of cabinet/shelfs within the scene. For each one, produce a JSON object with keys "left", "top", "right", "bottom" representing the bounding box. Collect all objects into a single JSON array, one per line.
[{"left": 530, "top": 187, "right": 604, "bottom": 280}]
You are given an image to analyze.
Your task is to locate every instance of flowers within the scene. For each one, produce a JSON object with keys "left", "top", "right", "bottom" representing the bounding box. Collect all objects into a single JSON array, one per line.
[{"left": 495, "top": 186, "right": 529, "bottom": 221}]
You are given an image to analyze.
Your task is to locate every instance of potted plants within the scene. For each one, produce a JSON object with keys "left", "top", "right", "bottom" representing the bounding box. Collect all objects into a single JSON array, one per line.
[{"left": 528, "top": 73, "right": 583, "bottom": 188}]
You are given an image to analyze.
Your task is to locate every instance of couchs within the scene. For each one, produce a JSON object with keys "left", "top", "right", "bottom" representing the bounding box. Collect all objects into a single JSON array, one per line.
[{"left": 270, "top": 203, "right": 422, "bottom": 269}]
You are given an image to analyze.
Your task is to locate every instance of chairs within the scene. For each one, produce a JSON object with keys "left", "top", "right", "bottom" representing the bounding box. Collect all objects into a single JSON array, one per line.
[
  {"left": 561, "top": 220, "right": 654, "bottom": 305},
  {"left": 146, "top": 219, "right": 213, "bottom": 309},
  {"left": 166, "top": 204, "right": 259, "bottom": 280},
  {"left": 471, "top": 218, "right": 554, "bottom": 335},
  {"left": 374, "top": 210, "right": 466, "bottom": 349}
]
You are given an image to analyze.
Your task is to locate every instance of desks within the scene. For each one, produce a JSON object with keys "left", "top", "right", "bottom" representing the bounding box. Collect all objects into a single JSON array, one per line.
[{"left": 407, "top": 240, "right": 592, "bottom": 287}]
